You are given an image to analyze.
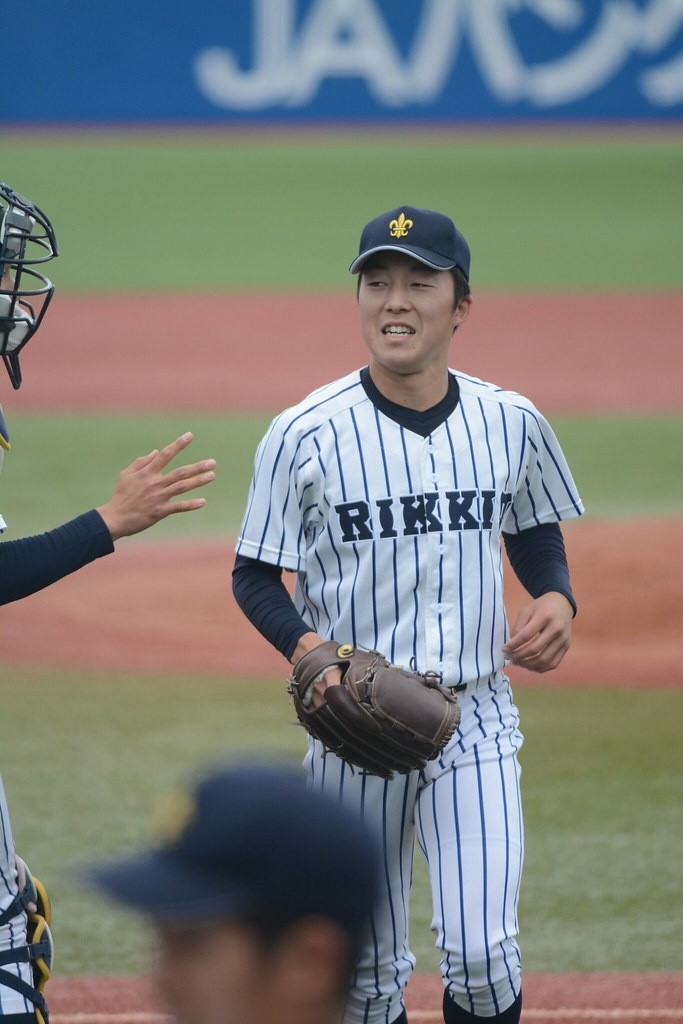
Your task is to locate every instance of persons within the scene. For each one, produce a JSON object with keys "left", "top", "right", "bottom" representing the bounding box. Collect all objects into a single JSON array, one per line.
[
  {"left": 230, "top": 204, "right": 585, "bottom": 1024},
  {"left": 0, "top": 183, "right": 218, "bottom": 1024},
  {"left": 71, "top": 766, "right": 385, "bottom": 1024}
]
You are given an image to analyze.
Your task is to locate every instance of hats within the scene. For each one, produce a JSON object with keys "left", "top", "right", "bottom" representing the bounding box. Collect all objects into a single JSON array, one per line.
[
  {"left": 349, "top": 206, "right": 470, "bottom": 282},
  {"left": 87, "top": 766, "right": 382, "bottom": 933}
]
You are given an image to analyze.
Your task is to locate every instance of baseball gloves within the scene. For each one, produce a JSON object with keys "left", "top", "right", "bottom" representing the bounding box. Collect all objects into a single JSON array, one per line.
[{"left": 287, "top": 638, "right": 463, "bottom": 781}]
